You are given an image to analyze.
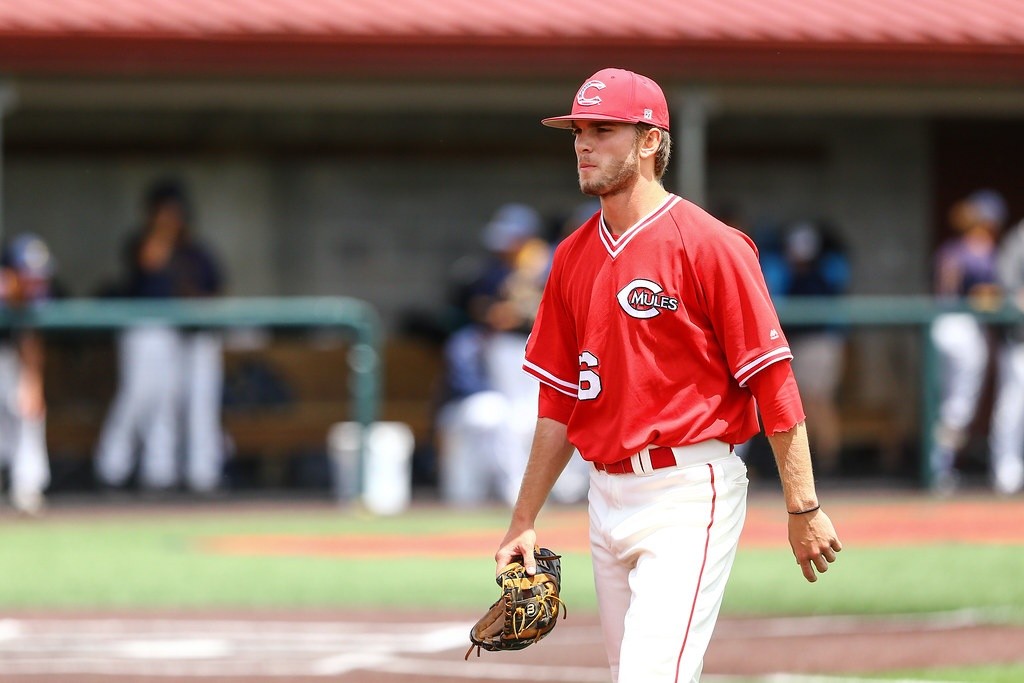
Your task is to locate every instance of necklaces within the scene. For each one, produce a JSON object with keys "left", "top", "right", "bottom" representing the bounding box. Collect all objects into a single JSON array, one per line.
[{"left": 612, "top": 234, "right": 621, "bottom": 240}]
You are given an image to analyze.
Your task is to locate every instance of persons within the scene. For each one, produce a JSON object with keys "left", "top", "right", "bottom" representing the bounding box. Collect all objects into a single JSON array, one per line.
[
  {"left": 440, "top": 204, "right": 600, "bottom": 510},
  {"left": 93, "top": 169, "right": 228, "bottom": 490},
  {"left": 495, "top": 68, "right": 842, "bottom": 683},
  {"left": 756, "top": 176, "right": 1024, "bottom": 502},
  {"left": 0, "top": 234, "right": 53, "bottom": 516}
]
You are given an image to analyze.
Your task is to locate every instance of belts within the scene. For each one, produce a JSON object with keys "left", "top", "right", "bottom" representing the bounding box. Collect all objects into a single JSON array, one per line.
[{"left": 594, "top": 443, "right": 734, "bottom": 474}]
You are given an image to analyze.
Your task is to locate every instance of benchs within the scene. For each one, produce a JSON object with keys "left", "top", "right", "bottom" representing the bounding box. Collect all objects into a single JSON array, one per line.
[{"left": 42, "top": 333, "right": 449, "bottom": 492}]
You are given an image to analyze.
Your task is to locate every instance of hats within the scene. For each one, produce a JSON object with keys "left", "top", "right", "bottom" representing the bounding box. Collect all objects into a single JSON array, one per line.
[
  {"left": 7, "top": 234, "right": 58, "bottom": 280},
  {"left": 541, "top": 68, "right": 670, "bottom": 132},
  {"left": 483, "top": 198, "right": 537, "bottom": 250}
]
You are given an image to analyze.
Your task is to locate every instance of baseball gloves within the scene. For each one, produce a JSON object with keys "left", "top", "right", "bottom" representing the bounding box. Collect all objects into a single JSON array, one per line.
[{"left": 464, "top": 544, "right": 568, "bottom": 662}]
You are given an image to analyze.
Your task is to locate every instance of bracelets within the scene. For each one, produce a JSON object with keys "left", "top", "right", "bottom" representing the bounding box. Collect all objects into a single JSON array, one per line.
[{"left": 787, "top": 505, "right": 820, "bottom": 515}]
